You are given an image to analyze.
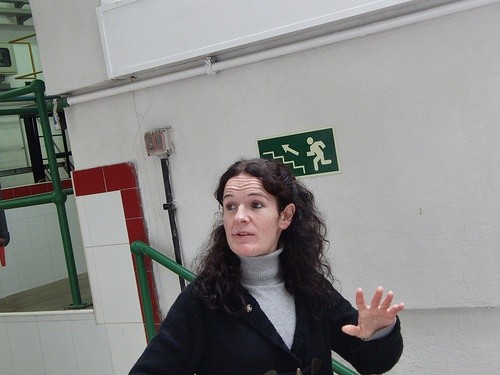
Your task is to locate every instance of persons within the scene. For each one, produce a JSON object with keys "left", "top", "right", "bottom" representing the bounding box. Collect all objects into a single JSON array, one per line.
[{"left": 127, "top": 159, "right": 404, "bottom": 375}]
